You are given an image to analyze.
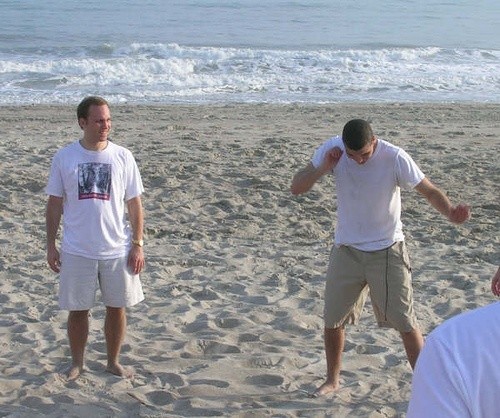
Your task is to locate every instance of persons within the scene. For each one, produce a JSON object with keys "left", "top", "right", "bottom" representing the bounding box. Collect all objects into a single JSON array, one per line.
[
  {"left": 290, "top": 118, "right": 471, "bottom": 395},
  {"left": 44, "top": 96, "right": 145, "bottom": 384},
  {"left": 406, "top": 258, "right": 500, "bottom": 418}
]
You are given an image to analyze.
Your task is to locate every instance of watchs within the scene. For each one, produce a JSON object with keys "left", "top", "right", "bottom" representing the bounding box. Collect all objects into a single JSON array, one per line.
[{"left": 130, "top": 237, "right": 143, "bottom": 247}]
